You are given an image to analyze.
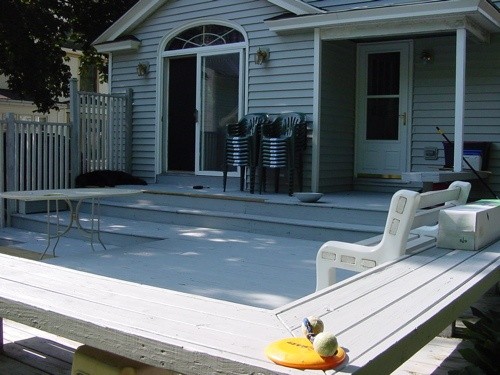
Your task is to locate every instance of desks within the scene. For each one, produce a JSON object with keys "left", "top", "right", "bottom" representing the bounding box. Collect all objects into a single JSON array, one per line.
[{"left": 0, "top": 186, "right": 143, "bottom": 260}]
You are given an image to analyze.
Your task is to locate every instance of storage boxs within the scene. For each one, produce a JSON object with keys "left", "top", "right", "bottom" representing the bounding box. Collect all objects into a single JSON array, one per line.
[{"left": 438, "top": 198, "right": 500, "bottom": 251}]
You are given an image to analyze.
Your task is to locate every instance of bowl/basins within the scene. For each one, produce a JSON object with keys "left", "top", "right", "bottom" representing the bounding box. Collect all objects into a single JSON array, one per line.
[{"left": 293, "top": 193, "right": 322, "bottom": 203}]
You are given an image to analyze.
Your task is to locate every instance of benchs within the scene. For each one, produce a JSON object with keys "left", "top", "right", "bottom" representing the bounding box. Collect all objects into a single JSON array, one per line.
[{"left": 315, "top": 181, "right": 472, "bottom": 291}]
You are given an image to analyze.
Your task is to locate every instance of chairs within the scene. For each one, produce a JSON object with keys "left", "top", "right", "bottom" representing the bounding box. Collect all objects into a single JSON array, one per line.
[{"left": 222, "top": 112, "right": 306, "bottom": 197}]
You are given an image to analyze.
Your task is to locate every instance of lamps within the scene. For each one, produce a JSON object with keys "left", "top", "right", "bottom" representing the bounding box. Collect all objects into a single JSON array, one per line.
[
  {"left": 137, "top": 62, "right": 149, "bottom": 77},
  {"left": 253, "top": 47, "right": 271, "bottom": 66}
]
